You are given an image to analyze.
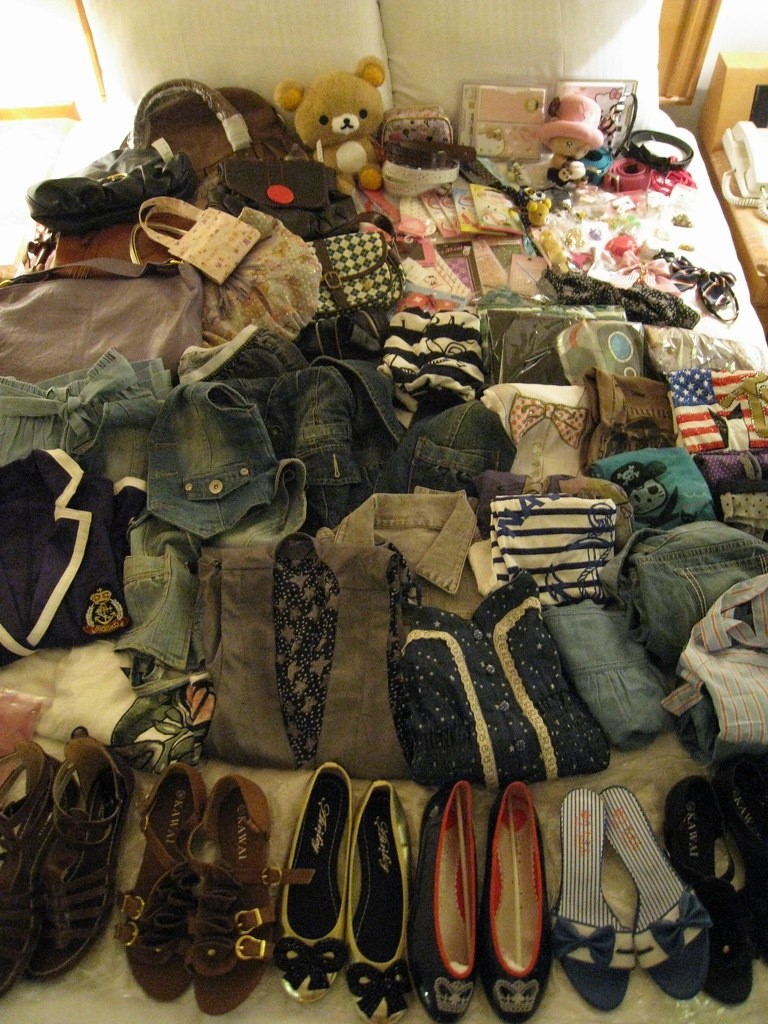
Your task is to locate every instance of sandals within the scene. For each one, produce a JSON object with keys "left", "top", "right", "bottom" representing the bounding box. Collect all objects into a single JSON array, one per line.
[
  {"left": 188, "top": 774, "right": 316, "bottom": 1015},
  {"left": 0, "top": 738, "right": 81, "bottom": 1004},
  {"left": 113, "top": 762, "right": 208, "bottom": 1002},
  {"left": 23, "top": 736, "right": 133, "bottom": 980}
]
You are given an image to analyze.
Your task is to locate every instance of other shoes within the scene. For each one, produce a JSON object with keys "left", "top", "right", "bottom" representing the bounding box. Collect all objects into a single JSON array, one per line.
[
  {"left": 272, "top": 761, "right": 353, "bottom": 1005},
  {"left": 405, "top": 779, "right": 482, "bottom": 1024},
  {"left": 344, "top": 778, "right": 410, "bottom": 1024},
  {"left": 479, "top": 781, "right": 549, "bottom": 1023}
]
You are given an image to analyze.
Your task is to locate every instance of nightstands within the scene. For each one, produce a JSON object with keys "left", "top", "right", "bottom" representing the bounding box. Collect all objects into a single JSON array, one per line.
[{"left": 694, "top": 51, "right": 768, "bottom": 344}]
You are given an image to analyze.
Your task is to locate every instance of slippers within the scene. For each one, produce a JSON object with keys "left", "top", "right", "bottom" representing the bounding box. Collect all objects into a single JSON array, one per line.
[
  {"left": 599, "top": 786, "right": 714, "bottom": 1000},
  {"left": 713, "top": 754, "right": 768, "bottom": 967},
  {"left": 662, "top": 776, "right": 755, "bottom": 1006},
  {"left": 549, "top": 789, "right": 635, "bottom": 1011}
]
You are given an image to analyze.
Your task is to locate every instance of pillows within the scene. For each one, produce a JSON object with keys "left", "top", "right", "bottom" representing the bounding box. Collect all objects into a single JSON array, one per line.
[
  {"left": 377, "top": 0, "right": 662, "bottom": 130},
  {"left": 83, "top": 0, "right": 393, "bottom": 132}
]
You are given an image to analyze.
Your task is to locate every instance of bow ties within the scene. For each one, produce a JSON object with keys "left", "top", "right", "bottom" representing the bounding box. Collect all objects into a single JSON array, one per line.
[{"left": 605, "top": 248, "right": 741, "bottom": 322}]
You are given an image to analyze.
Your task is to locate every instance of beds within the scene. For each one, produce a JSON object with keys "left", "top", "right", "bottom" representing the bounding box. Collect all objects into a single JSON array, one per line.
[{"left": 0, "top": 1, "right": 768, "bottom": 1022}]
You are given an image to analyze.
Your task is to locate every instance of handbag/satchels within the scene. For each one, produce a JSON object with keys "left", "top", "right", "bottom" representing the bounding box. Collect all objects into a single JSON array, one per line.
[
  {"left": 138, "top": 198, "right": 262, "bottom": 284},
  {"left": 302, "top": 211, "right": 407, "bottom": 317},
  {"left": 217, "top": 160, "right": 359, "bottom": 243},
  {"left": 24, "top": 136, "right": 199, "bottom": 236},
  {"left": 129, "top": 207, "right": 322, "bottom": 342},
  {"left": 380, "top": 105, "right": 454, "bottom": 147}
]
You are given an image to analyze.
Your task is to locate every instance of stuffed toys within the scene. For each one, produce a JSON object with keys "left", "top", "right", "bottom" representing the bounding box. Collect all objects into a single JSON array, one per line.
[
  {"left": 274, "top": 55, "right": 386, "bottom": 196},
  {"left": 541, "top": 95, "right": 604, "bottom": 192},
  {"left": 525, "top": 198, "right": 551, "bottom": 227}
]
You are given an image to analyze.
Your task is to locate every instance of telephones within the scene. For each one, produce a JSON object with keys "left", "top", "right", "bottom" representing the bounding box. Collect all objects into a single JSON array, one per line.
[{"left": 721, "top": 120, "right": 768, "bottom": 199}]
